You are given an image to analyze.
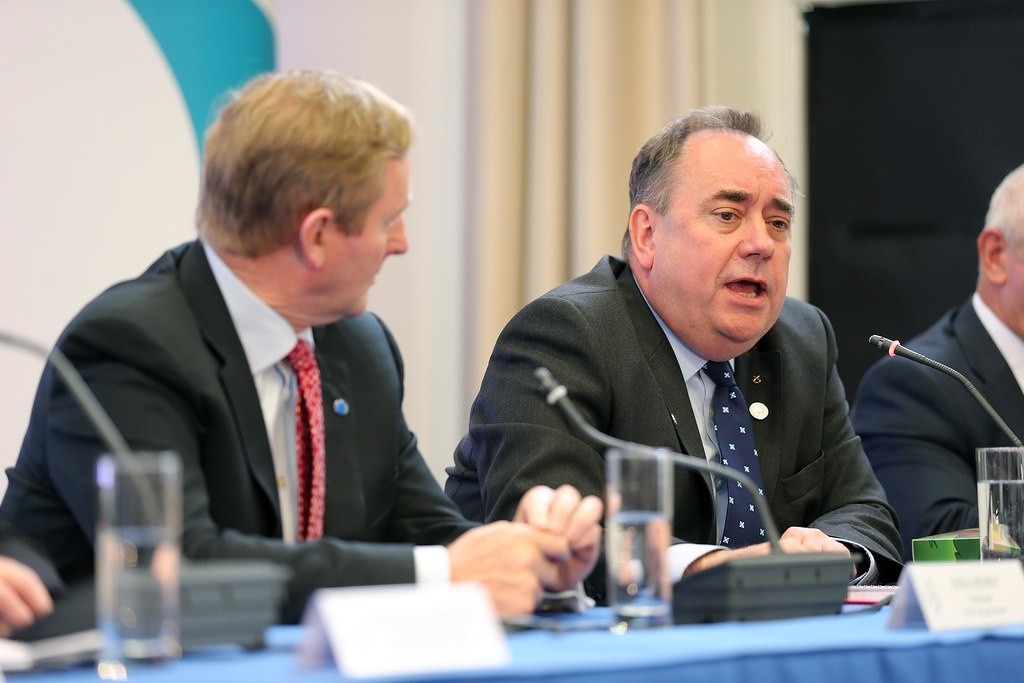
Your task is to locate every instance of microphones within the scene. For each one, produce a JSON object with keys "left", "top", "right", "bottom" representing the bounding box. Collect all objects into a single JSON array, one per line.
[
  {"left": 532, "top": 365, "right": 862, "bottom": 628},
  {"left": 869, "top": 335, "right": 1021, "bottom": 447}
]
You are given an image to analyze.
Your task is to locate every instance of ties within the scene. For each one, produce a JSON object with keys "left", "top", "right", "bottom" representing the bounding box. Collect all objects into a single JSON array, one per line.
[
  {"left": 701, "top": 359, "right": 767, "bottom": 547},
  {"left": 286, "top": 339, "right": 325, "bottom": 543}
]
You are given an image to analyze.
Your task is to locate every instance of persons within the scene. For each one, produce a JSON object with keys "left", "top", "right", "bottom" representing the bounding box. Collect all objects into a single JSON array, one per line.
[
  {"left": 852, "top": 166, "right": 1024, "bottom": 561},
  {"left": 443, "top": 106, "right": 903, "bottom": 603},
  {"left": 0, "top": 555, "right": 55, "bottom": 637},
  {"left": 0, "top": 71, "right": 602, "bottom": 625}
]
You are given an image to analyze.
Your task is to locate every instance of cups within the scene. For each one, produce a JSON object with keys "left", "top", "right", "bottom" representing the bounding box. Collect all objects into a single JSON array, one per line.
[
  {"left": 976, "top": 446, "right": 1024, "bottom": 562},
  {"left": 94, "top": 451, "right": 184, "bottom": 681},
  {"left": 605, "top": 448, "right": 674, "bottom": 619}
]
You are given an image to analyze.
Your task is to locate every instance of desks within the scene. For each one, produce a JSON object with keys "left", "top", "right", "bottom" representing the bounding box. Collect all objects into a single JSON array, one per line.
[{"left": 0, "top": 584, "right": 1024, "bottom": 683}]
